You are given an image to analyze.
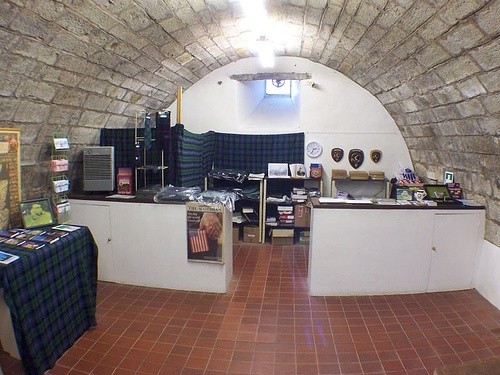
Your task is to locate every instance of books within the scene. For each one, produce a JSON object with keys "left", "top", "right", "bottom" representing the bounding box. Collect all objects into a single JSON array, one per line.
[
  {"left": 0, "top": 251, "right": 20, "bottom": 264},
  {"left": 0, "top": 224, "right": 81, "bottom": 250}
]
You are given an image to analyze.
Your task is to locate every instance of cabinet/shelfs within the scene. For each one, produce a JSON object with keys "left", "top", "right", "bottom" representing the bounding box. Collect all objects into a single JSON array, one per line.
[
  {"left": 205, "top": 174, "right": 323, "bottom": 243},
  {"left": 51, "top": 134, "right": 72, "bottom": 225},
  {"left": 308, "top": 196, "right": 486, "bottom": 296},
  {"left": 135, "top": 110, "right": 172, "bottom": 190},
  {"left": 331, "top": 178, "right": 390, "bottom": 199}
]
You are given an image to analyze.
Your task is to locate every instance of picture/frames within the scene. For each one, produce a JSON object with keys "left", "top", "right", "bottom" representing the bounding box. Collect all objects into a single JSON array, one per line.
[
  {"left": 268, "top": 164, "right": 288, "bottom": 178},
  {"left": 18, "top": 197, "right": 56, "bottom": 230},
  {"left": 423, "top": 184, "right": 452, "bottom": 200}
]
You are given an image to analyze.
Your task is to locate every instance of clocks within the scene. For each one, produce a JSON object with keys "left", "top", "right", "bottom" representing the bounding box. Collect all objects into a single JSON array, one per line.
[{"left": 306, "top": 142, "right": 323, "bottom": 158}]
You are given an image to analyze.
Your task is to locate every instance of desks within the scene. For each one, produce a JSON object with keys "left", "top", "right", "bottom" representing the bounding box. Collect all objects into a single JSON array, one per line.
[
  {"left": 0, "top": 224, "right": 98, "bottom": 375},
  {"left": 67, "top": 189, "right": 232, "bottom": 294}
]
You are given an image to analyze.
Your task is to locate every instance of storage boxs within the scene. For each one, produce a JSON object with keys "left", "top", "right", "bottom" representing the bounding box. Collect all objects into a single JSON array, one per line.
[
  {"left": 294, "top": 204, "right": 311, "bottom": 228},
  {"left": 332, "top": 169, "right": 384, "bottom": 180},
  {"left": 268, "top": 228, "right": 294, "bottom": 246},
  {"left": 232, "top": 224, "right": 259, "bottom": 242}
]
